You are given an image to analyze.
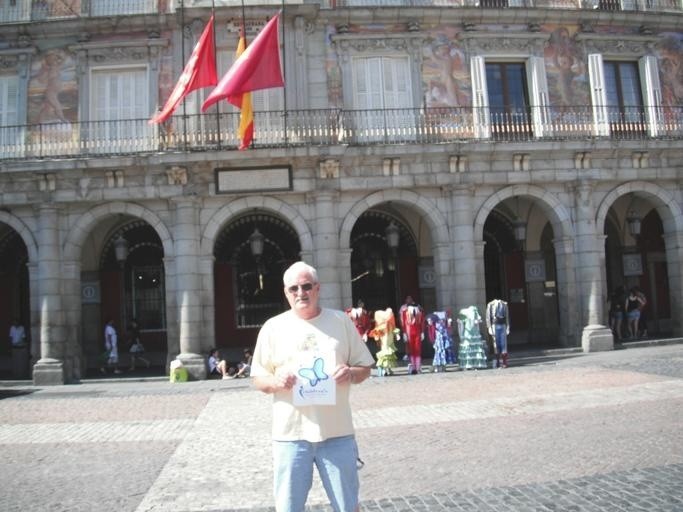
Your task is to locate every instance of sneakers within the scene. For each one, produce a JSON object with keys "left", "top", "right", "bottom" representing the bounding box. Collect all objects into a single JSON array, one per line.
[
  {"left": 222, "top": 372, "right": 246, "bottom": 380},
  {"left": 378, "top": 365, "right": 488, "bottom": 376},
  {"left": 113, "top": 360, "right": 153, "bottom": 375}
]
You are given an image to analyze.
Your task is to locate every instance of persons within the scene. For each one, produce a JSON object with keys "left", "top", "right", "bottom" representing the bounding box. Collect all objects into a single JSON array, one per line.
[
  {"left": 9, "top": 317, "right": 26, "bottom": 346},
  {"left": 233, "top": 348, "right": 253, "bottom": 378},
  {"left": 343, "top": 297, "right": 511, "bottom": 376},
  {"left": 608, "top": 285, "right": 650, "bottom": 341},
  {"left": 121, "top": 318, "right": 151, "bottom": 371},
  {"left": 207, "top": 348, "right": 233, "bottom": 379},
  {"left": 99, "top": 315, "right": 123, "bottom": 375},
  {"left": 250, "top": 261, "right": 375, "bottom": 512}
]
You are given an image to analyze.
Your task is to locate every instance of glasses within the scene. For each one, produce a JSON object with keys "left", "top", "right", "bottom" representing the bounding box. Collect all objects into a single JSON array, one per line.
[{"left": 286, "top": 282, "right": 319, "bottom": 292}]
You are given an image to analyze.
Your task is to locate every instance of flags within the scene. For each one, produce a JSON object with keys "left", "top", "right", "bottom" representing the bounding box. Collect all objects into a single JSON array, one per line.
[
  {"left": 225, "top": 16, "right": 254, "bottom": 151},
  {"left": 200, "top": 8, "right": 285, "bottom": 112},
  {"left": 146, "top": 15, "right": 218, "bottom": 124}
]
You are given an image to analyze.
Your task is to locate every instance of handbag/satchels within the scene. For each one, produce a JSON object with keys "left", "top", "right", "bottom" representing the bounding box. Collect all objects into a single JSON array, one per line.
[
  {"left": 95, "top": 343, "right": 113, "bottom": 369},
  {"left": 128, "top": 337, "right": 144, "bottom": 353}
]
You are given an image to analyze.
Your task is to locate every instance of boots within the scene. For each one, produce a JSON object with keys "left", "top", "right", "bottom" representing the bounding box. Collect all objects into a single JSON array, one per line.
[
  {"left": 502, "top": 352, "right": 508, "bottom": 367},
  {"left": 493, "top": 354, "right": 500, "bottom": 368}
]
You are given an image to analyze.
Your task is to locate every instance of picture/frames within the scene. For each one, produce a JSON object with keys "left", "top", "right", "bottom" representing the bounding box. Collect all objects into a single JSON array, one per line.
[{"left": 213, "top": 165, "right": 294, "bottom": 194}]
